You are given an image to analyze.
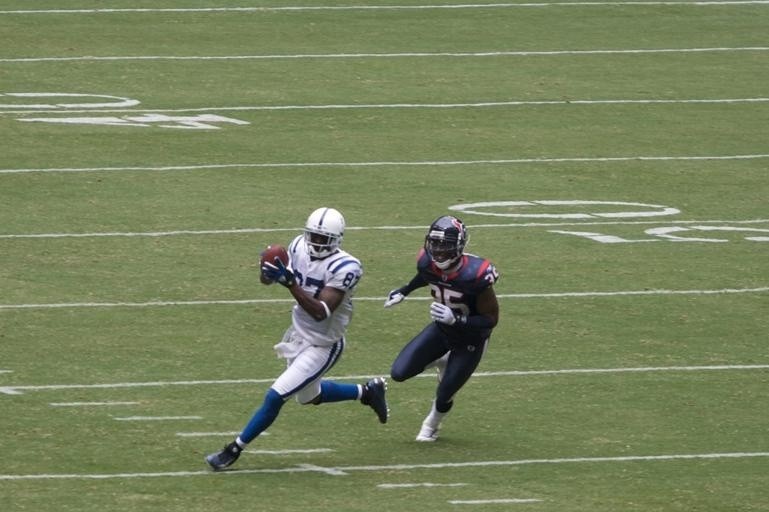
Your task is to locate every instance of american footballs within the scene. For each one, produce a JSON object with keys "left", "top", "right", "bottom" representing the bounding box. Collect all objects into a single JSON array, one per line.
[{"left": 260, "top": 245, "right": 289, "bottom": 275}]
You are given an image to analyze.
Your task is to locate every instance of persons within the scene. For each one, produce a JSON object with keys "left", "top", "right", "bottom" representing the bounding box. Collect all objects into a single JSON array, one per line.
[
  {"left": 383, "top": 215, "right": 499, "bottom": 442},
  {"left": 205, "top": 208, "right": 387, "bottom": 470}
]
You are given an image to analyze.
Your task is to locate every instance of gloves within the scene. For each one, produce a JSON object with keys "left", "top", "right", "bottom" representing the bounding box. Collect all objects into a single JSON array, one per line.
[
  {"left": 430, "top": 301, "right": 457, "bottom": 326},
  {"left": 259, "top": 246, "right": 295, "bottom": 287},
  {"left": 384, "top": 289, "right": 405, "bottom": 308}
]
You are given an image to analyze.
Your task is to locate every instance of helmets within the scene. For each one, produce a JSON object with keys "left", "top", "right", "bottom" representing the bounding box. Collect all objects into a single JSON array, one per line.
[
  {"left": 303, "top": 207, "right": 345, "bottom": 237},
  {"left": 426, "top": 215, "right": 467, "bottom": 243}
]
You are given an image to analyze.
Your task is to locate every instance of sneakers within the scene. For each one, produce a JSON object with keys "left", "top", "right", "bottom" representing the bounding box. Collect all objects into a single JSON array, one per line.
[
  {"left": 415, "top": 423, "right": 441, "bottom": 442},
  {"left": 205, "top": 441, "right": 241, "bottom": 469},
  {"left": 365, "top": 377, "right": 390, "bottom": 424},
  {"left": 438, "top": 350, "right": 451, "bottom": 383}
]
